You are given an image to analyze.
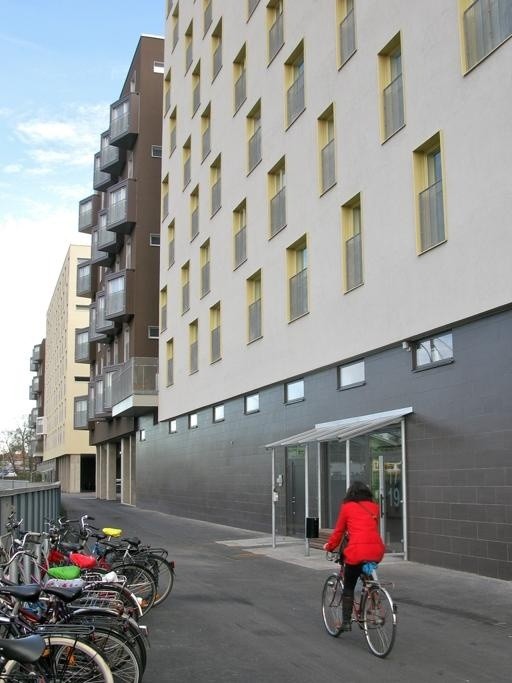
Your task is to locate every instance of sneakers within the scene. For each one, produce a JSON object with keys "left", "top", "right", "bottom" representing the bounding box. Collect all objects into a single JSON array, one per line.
[{"left": 337, "top": 623, "right": 352, "bottom": 631}]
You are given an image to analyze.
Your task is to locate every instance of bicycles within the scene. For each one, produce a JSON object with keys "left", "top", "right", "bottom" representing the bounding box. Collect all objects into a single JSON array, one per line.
[
  {"left": 0, "top": 506, "right": 176, "bottom": 683},
  {"left": 322, "top": 551, "right": 397, "bottom": 657}
]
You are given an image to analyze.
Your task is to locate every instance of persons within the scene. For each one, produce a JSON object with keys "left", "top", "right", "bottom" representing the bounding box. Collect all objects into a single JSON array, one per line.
[{"left": 323, "top": 481, "right": 385, "bottom": 632}]
